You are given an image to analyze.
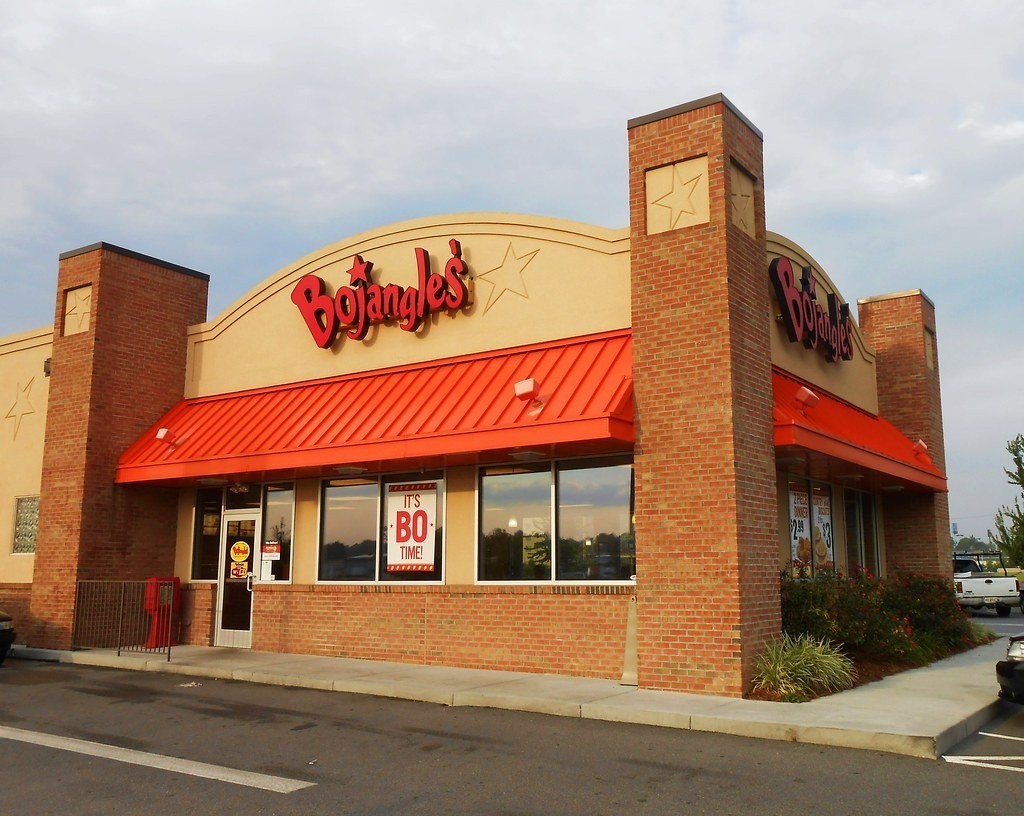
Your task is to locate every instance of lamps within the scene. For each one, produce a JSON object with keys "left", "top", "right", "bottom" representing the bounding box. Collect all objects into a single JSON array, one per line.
[
  {"left": 514, "top": 378, "right": 543, "bottom": 406},
  {"left": 156, "top": 428, "right": 177, "bottom": 445},
  {"left": 795, "top": 386, "right": 820, "bottom": 417},
  {"left": 913, "top": 439, "right": 927, "bottom": 458}
]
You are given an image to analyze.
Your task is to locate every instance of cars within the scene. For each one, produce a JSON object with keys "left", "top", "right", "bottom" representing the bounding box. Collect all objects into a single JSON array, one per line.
[
  {"left": 0, "top": 609, "right": 18, "bottom": 665},
  {"left": 995, "top": 631, "right": 1024, "bottom": 705}
]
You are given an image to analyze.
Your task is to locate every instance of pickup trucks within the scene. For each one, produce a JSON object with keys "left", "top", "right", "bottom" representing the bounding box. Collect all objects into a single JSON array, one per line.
[{"left": 952, "top": 551, "right": 1021, "bottom": 618}]
